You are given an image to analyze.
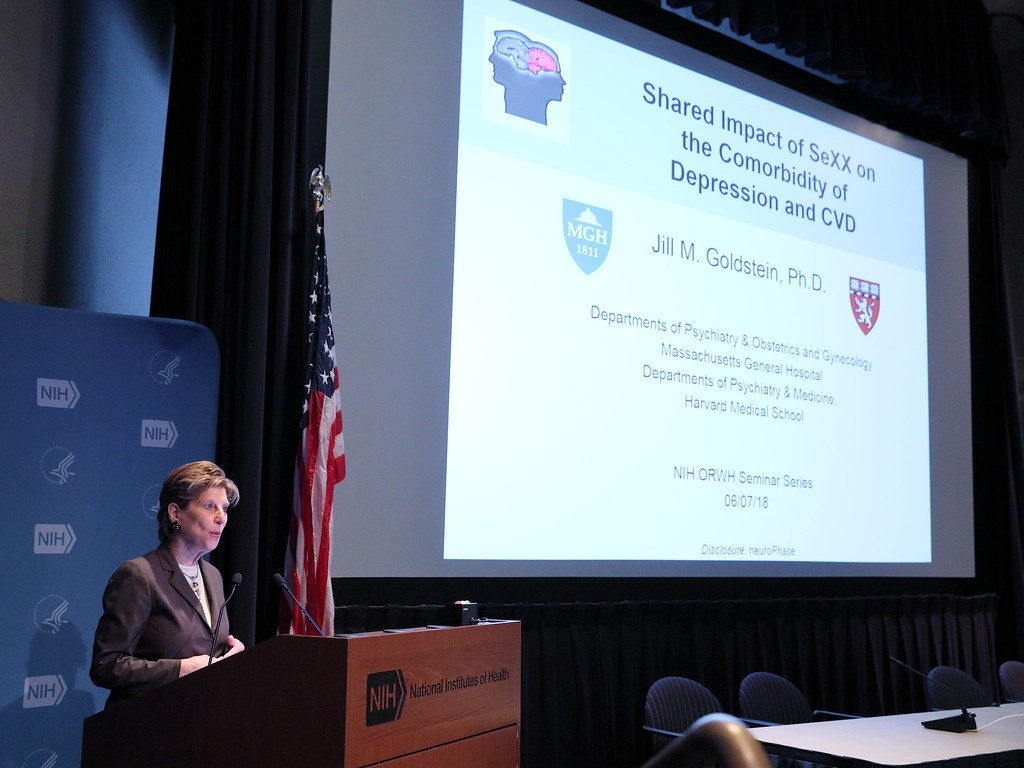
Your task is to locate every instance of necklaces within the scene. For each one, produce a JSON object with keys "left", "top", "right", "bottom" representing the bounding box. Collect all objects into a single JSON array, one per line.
[{"left": 183, "top": 562, "right": 199, "bottom": 580}]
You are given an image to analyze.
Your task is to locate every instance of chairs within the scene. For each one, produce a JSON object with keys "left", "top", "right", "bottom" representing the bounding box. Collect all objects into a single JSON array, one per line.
[
  {"left": 739, "top": 672, "right": 862, "bottom": 768},
  {"left": 642, "top": 678, "right": 723, "bottom": 755},
  {"left": 927, "top": 666, "right": 994, "bottom": 713},
  {"left": 999, "top": 661, "right": 1024, "bottom": 704}
]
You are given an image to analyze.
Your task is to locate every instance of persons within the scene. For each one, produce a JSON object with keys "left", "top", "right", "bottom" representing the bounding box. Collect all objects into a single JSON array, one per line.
[{"left": 90, "top": 460, "right": 246, "bottom": 710}]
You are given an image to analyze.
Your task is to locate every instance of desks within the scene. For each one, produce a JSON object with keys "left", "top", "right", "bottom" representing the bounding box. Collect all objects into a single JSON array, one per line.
[{"left": 746, "top": 702, "right": 1024, "bottom": 768}]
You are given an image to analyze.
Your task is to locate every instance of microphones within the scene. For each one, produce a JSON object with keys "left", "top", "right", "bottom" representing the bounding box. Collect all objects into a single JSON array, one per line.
[
  {"left": 889, "top": 655, "right": 977, "bottom": 733},
  {"left": 273, "top": 572, "right": 325, "bottom": 636},
  {"left": 207, "top": 572, "right": 243, "bottom": 666}
]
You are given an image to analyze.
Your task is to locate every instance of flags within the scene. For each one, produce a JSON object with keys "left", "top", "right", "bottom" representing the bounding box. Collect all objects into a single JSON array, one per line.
[{"left": 276, "top": 188, "right": 347, "bottom": 637}]
